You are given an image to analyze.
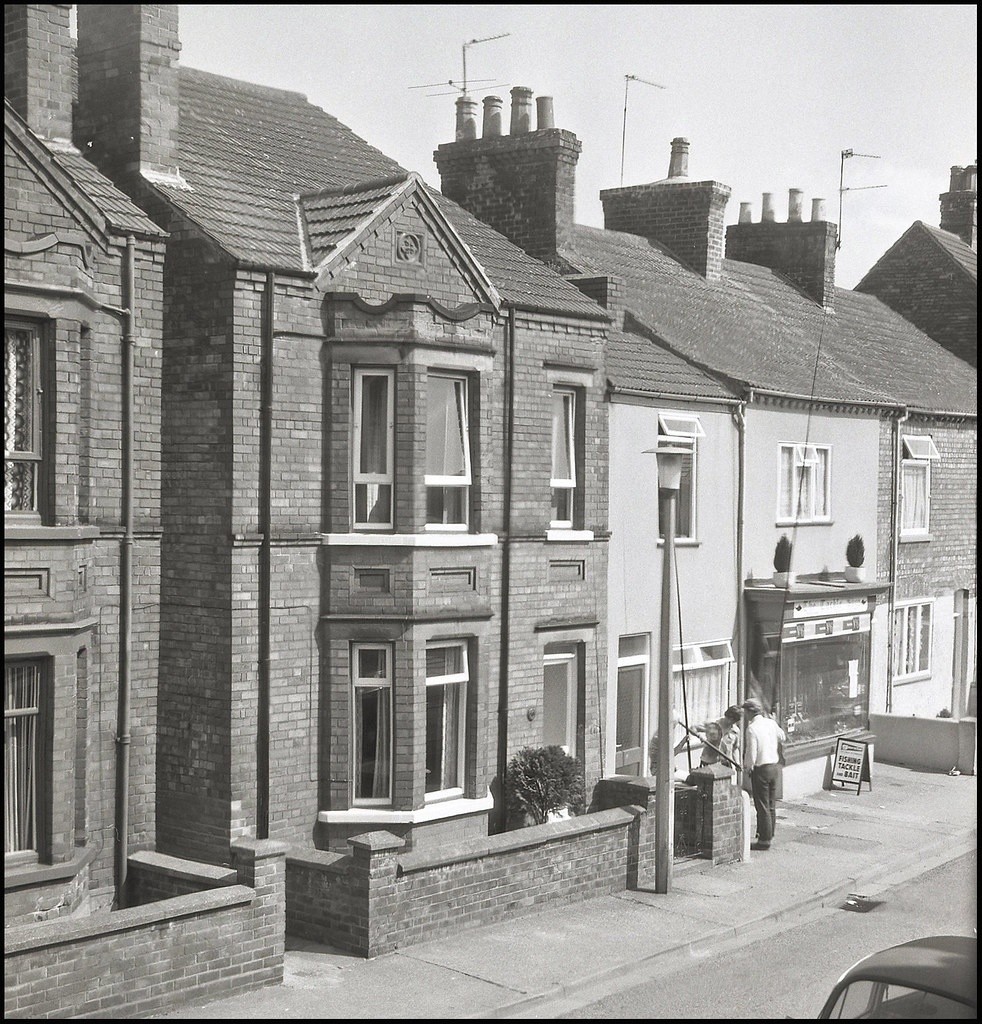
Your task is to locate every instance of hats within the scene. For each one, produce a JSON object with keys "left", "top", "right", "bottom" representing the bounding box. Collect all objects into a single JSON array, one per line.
[{"left": 742, "top": 698, "right": 762, "bottom": 711}]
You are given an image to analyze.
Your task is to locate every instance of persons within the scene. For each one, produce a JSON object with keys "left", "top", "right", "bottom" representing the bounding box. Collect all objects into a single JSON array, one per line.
[
  {"left": 650, "top": 710, "right": 691, "bottom": 782},
  {"left": 742, "top": 698, "right": 786, "bottom": 851},
  {"left": 689, "top": 705, "right": 742, "bottom": 768}
]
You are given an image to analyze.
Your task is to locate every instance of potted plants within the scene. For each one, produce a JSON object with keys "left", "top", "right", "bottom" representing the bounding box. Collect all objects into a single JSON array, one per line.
[
  {"left": 773, "top": 532, "right": 795, "bottom": 588},
  {"left": 844, "top": 533, "right": 865, "bottom": 583}
]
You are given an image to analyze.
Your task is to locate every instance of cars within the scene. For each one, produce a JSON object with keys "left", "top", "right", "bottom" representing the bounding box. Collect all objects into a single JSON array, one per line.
[{"left": 815, "top": 933, "right": 977, "bottom": 1019}]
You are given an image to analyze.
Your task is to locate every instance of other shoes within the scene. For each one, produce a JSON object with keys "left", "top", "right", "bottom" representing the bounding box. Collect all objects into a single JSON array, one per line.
[{"left": 750, "top": 841, "right": 770, "bottom": 850}]
